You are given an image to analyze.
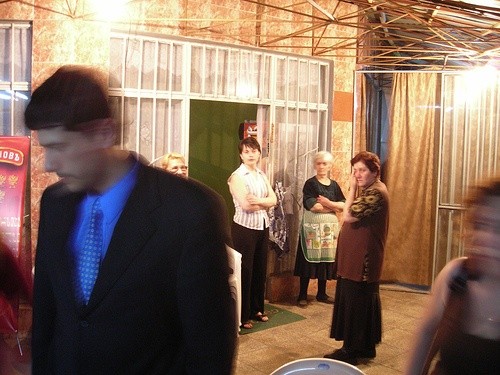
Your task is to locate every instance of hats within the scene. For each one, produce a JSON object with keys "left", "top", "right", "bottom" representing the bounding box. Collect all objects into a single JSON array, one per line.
[{"left": 24, "top": 72, "right": 112, "bottom": 130}]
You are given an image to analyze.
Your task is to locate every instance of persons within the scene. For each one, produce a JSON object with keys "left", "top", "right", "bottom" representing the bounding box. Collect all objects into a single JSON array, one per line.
[
  {"left": 162, "top": 152, "right": 188, "bottom": 180},
  {"left": 294, "top": 151, "right": 346, "bottom": 309},
  {"left": 225, "top": 136, "right": 277, "bottom": 328},
  {"left": 400, "top": 175, "right": 500, "bottom": 375},
  {"left": 324, "top": 152, "right": 391, "bottom": 366},
  {"left": 22, "top": 64, "right": 238, "bottom": 375}
]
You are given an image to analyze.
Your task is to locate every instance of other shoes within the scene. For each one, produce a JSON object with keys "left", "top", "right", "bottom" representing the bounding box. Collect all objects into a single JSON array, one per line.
[
  {"left": 297, "top": 298, "right": 308, "bottom": 309},
  {"left": 316, "top": 294, "right": 334, "bottom": 304},
  {"left": 254, "top": 315, "right": 269, "bottom": 322},
  {"left": 241, "top": 320, "right": 254, "bottom": 329},
  {"left": 324, "top": 349, "right": 371, "bottom": 365}
]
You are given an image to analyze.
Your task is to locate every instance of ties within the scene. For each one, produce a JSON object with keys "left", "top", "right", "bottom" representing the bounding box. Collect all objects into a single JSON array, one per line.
[{"left": 68, "top": 200, "right": 103, "bottom": 307}]
